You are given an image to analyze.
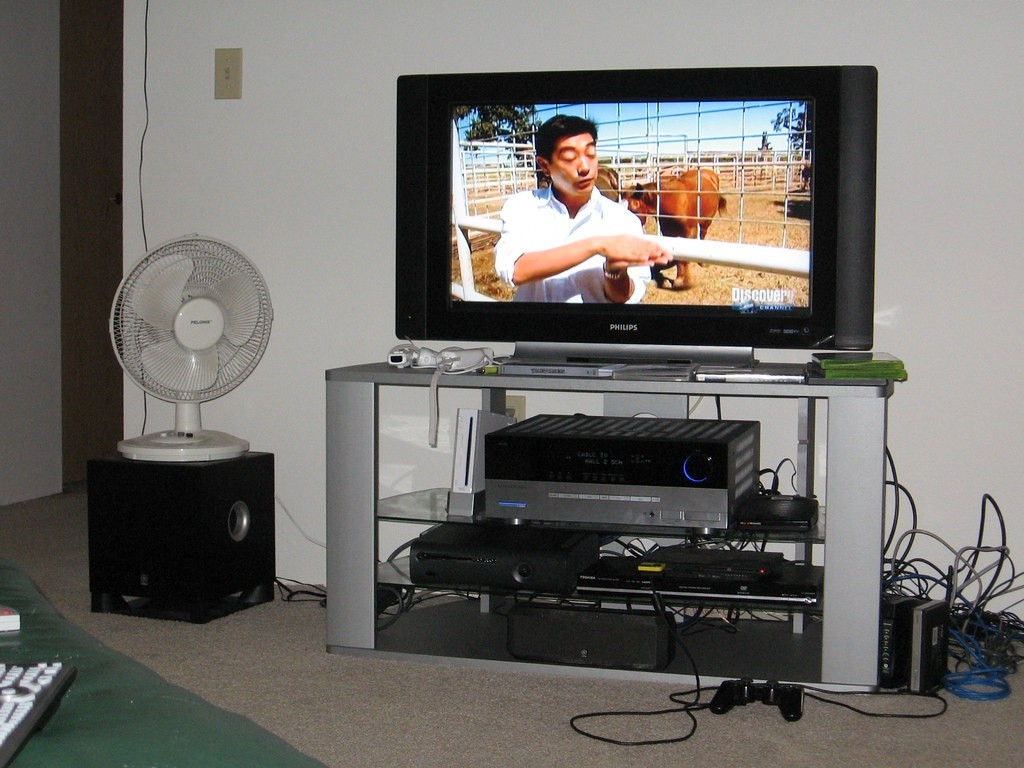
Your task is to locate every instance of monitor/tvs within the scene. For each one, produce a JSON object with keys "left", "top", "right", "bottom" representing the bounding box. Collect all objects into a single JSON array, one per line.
[{"left": 392, "top": 65, "right": 880, "bottom": 378}]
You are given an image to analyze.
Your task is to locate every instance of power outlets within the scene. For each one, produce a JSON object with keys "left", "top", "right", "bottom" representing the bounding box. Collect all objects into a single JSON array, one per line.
[{"left": 505, "top": 395, "right": 526, "bottom": 423}]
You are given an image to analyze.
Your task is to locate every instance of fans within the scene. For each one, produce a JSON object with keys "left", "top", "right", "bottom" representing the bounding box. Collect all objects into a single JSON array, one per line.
[{"left": 107, "top": 233, "right": 275, "bottom": 461}]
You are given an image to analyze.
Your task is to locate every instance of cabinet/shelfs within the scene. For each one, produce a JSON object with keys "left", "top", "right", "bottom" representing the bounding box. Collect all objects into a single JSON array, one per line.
[{"left": 323, "top": 363, "right": 895, "bottom": 696}]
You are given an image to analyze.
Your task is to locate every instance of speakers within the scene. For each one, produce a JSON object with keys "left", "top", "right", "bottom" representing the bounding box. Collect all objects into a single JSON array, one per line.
[{"left": 83, "top": 450, "right": 276, "bottom": 623}]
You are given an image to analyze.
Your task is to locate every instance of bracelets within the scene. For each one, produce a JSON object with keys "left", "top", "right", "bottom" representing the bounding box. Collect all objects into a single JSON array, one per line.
[{"left": 603, "top": 262, "right": 627, "bottom": 279}]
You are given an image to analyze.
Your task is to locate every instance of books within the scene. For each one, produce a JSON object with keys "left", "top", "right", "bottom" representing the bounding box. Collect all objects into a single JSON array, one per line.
[{"left": 806, "top": 353, "right": 908, "bottom": 385}]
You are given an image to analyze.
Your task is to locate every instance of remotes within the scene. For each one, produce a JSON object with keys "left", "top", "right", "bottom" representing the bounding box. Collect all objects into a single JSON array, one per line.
[{"left": 0, "top": 662, "right": 80, "bottom": 768}]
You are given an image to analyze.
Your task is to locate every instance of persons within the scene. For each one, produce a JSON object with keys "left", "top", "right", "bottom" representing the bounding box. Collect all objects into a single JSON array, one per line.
[{"left": 493, "top": 113, "right": 673, "bottom": 305}]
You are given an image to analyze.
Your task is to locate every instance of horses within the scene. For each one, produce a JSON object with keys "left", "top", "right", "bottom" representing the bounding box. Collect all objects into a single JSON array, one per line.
[
  {"left": 538, "top": 164, "right": 619, "bottom": 202},
  {"left": 623, "top": 168, "right": 728, "bottom": 289}
]
